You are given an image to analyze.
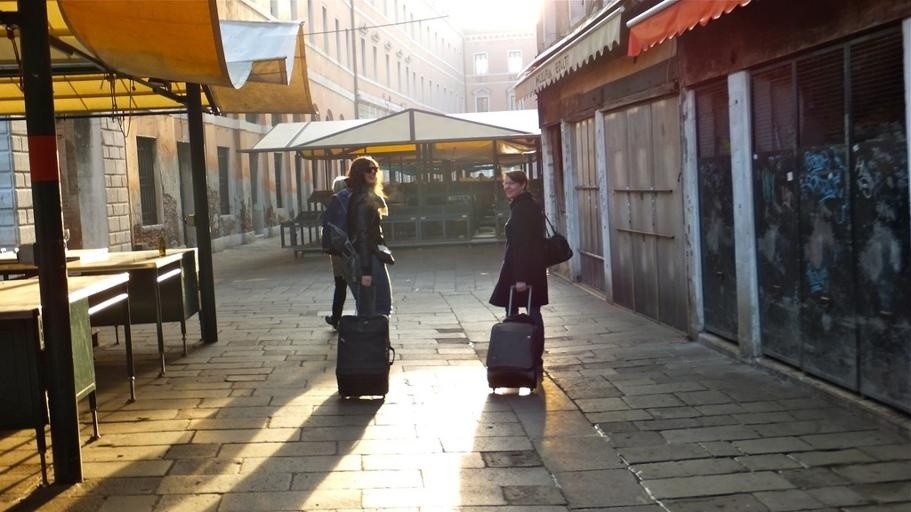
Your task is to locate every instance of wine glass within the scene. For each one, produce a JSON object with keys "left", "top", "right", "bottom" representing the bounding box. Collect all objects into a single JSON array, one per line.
[{"left": 64, "top": 227, "right": 70, "bottom": 251}]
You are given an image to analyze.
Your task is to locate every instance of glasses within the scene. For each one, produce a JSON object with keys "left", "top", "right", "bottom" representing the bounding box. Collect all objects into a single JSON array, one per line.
[
  {"left": 502, "top": 182, "right": 518, "bottom": 186},
  {"left": 363, "top": 166, "right": 378, "bottom": 174}
]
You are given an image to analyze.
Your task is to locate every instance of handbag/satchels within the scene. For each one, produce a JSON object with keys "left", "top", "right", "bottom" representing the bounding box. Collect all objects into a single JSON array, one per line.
[
  {"left": 324, "top": 221, "right": 355, "bottom": 259},
  {"left": 538, "top": 203, "right": 573, "bottom": 269},
  {"left": 373, "top": 242, "right": 395, "bottom": 265}
]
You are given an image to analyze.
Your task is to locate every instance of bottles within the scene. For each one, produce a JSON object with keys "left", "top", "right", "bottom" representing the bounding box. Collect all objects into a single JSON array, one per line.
[{"left": 159, "top": 237, "right": 166, "bottom": 257}]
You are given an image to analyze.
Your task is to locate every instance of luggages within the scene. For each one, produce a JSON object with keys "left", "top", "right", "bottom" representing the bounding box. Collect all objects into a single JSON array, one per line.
[
  {"left": 334, "top": 278, "right": 395, "bottom": 400},
  {"left": 484, "top": 283, "right": 540, "bottom": 394}
]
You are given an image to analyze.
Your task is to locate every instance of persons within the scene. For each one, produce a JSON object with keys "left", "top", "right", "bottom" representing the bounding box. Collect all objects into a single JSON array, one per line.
[
  {"left": 346, "top": 153, "right": 393, "bottom": 317},
  {"left": 487, "top": 170, "right": 549, "bottom": 383},
  {"left": 321, "top": 175, "right": 348, "bottom": 326}
]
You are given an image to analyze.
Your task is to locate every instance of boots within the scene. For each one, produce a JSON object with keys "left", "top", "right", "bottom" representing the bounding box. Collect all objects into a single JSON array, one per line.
[{"left": 324, "top": 285, "right": 346, "bottom": 330}]
